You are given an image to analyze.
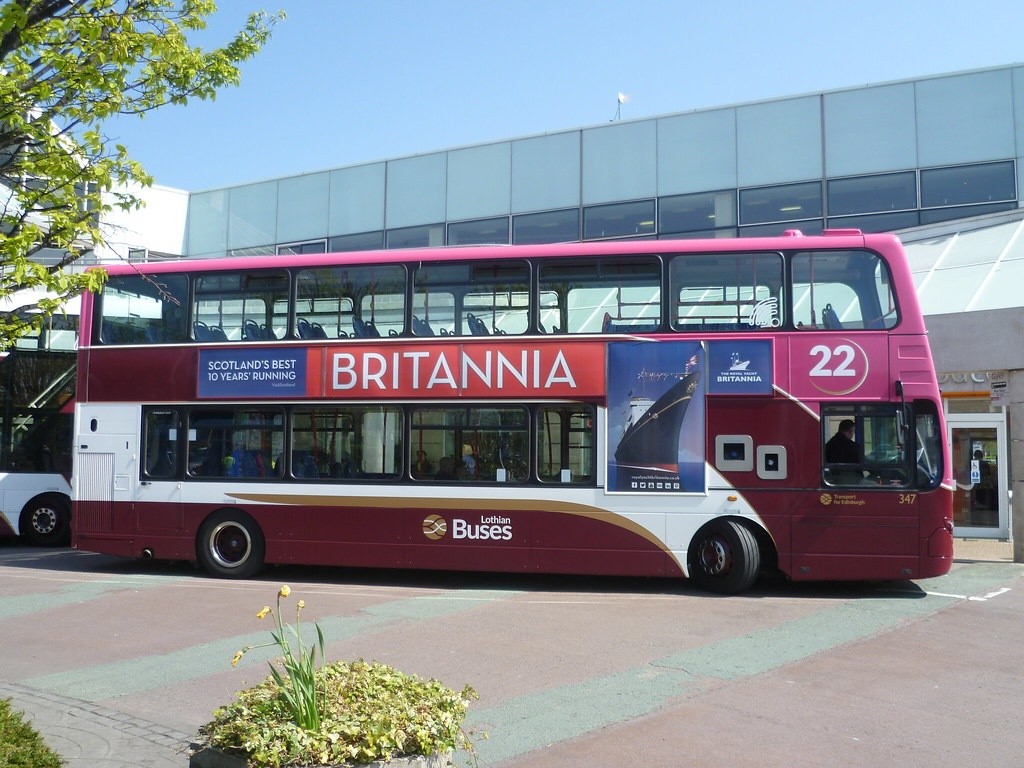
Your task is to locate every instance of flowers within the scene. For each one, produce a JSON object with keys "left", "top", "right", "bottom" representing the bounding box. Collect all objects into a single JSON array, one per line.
[{"left": 229, "top": 584, "right": 328, "bottom": 730}]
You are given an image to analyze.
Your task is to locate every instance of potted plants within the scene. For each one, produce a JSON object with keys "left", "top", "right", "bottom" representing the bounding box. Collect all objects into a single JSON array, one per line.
[{"left": 188, "top": 658, "right": 487, "bottom": 768}]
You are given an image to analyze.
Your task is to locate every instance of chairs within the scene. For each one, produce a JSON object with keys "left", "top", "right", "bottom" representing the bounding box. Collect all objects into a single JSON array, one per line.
[
  {"left": 101, "top": 313, "right": 507, "bottom": 346},
  {"left": 226, "top": 449, "right": 355, "bottom": 478},
  {"left": 528, "top": 311, "right": 568, "bottom": 335},
  {"left": 823, "top": 303, "right": 843, "bottom": 329}
]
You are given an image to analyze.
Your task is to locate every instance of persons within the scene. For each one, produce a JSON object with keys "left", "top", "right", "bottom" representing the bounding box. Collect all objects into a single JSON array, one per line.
[
  {"left": 972, "top": 450, "right": 992, "bottom": 509},
  {"left": 824, "top": 419, "right": 860, "bottom": 466}
]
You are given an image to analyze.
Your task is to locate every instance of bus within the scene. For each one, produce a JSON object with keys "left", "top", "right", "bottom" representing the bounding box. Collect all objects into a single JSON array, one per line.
[
  {"left": 70, "top": 224, "right": 959, "bottom": 593},
  {"left": 0, "top": 314, "right": 81, "bottom": 549}
]
